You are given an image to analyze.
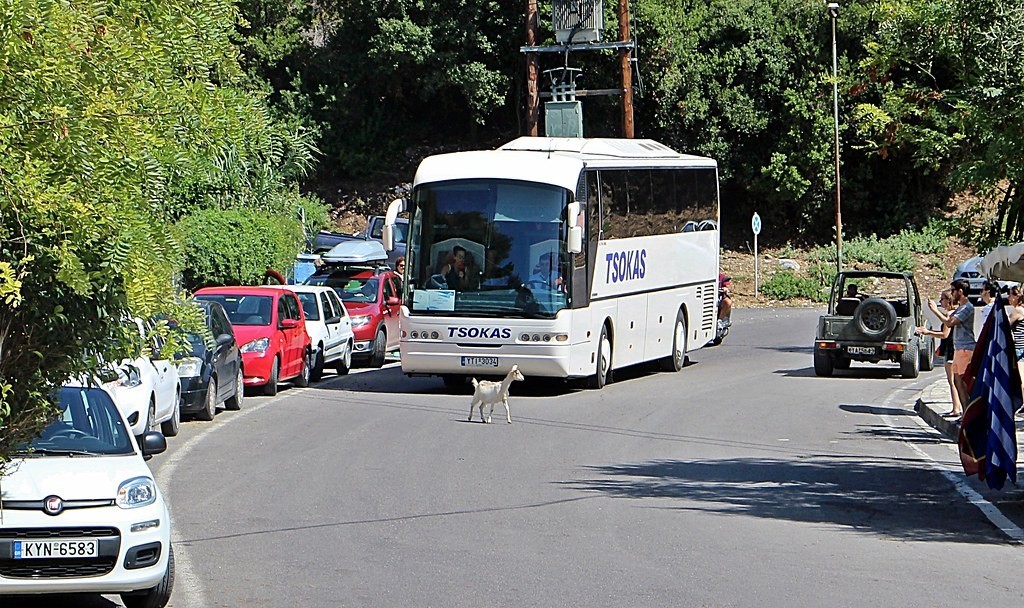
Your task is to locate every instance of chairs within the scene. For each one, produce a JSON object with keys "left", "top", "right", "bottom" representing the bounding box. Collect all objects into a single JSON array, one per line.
[
  {"left": 305, "top": 300, "right": 317, "bottom": 319},
  {"left": 258, "top": 297, "right": 270, "bottom": 321},
  {"left": 890, "top": 300, "right": 909, "bottom": 318},
  {"left": 840, "top": 298, "right": 861, "bottom": 315}
]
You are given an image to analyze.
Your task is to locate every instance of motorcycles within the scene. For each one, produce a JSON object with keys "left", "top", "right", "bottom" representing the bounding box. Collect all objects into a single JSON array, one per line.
[{"left": 709, "top": 276, "right": 732, "bottom": 346}]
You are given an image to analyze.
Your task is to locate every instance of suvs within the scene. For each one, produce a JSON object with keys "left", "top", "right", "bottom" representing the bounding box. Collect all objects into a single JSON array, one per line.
[{"left": 810, "top": 271, "right": 937, "bottom": 378}]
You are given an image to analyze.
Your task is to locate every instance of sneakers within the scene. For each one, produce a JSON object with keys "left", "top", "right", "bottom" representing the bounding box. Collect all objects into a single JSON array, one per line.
[{"left": 717, "top": 319, "right": 723, "bottom": 330}]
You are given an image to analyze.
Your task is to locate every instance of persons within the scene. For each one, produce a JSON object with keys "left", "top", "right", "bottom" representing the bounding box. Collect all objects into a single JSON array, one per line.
[
  {"left": 314, "top": 258, "right": 327, "bottom": 270},
  {"left": 718, "top": 266, "right": 732, "bottom": 330},
  {"left": 928, "top": 279, "right": 976, "bottom": 422},
  {"left": 847, "top": 284, "right": 869, "bottom": 302},
  {"left": 440, "top": 245, "right": 470, "bottom": 291},
  {"left": 529, "top": 254, "right": 563, "bottom": 290},
  {"left": 979, "top": 281, "right": 1010, "bottom": 333},
  {"left": 394, "top": 257, "right": 405, "bottom": 283},
  {"left": 915, "top": 290, "right": 962, "bottom": 418},
  {"left": 1008, "top": 286, "right": 1024, "bottom": 414}
]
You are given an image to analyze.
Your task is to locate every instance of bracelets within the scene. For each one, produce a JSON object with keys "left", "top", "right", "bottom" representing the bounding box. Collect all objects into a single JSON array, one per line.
[{"left": 928, "top": 332, "right": 931, "bottom": 335}]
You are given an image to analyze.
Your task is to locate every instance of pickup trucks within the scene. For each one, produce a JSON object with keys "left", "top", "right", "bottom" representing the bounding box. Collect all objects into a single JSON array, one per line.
[{"left": 308, "top": 215, "right": 461, "bottom": 274}]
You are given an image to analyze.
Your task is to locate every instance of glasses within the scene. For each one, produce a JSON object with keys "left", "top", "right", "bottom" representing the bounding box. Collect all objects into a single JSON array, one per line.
[
  {"left": 397, "top": 264, "right": 405, "bottom": 267},
  {"left": 458, "top": 255, "right": 467, "bottom": 259},
  {"left": 951, "top": 289, "right": 959, "bottom": 290},
  {"left": 941, "top": 297, "right": 948, "bottom": 299},
  {"left": 539, "top": 262, "right": 551, "bottom": 266}
]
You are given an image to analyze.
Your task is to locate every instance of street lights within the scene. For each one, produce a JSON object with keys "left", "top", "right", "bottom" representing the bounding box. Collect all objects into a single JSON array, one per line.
[{"left": 825, "top": 1, "right": 854, "bottom": 272}]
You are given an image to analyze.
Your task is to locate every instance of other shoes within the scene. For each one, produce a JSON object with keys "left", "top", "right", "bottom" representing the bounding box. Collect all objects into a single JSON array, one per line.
[{"left": 942, "top": 411, "right": 963, "bottom": 417}]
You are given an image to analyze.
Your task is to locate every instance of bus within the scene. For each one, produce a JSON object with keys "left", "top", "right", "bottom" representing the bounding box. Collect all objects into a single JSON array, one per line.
[{"left": 395, "top": 135, "right": 716, "bottom": 388}]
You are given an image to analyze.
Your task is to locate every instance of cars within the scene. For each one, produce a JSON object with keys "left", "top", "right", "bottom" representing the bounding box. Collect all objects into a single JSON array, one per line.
[
  {"left": 183, "top": 286, "right": 311, "bottom": 396},
  {"left": 953, "top": 252, "right": 1022, "bottom": 304},
  {"left": 260, "top": 285, "right": 355, "bottom": 384},
  {"left": 0, "top": 374, "right": 176, "bottom": 608},
  {"left": 125, "top": 298, "right": 245, "bottom": 421},
  {"left": 48, "top": 315, "right": 181, "bottom": 457},
  {"left": 261, "top": 264, "right": 405, "bottom": 368}
]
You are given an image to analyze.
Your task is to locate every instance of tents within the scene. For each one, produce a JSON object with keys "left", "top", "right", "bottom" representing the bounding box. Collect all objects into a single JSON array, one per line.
[{"left": 978, "top": 241, "right": 1024, "bottom": 283}]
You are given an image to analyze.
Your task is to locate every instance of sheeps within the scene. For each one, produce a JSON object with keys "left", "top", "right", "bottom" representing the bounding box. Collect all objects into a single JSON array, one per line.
[{"left": 467, "top": 364, "right": 525, "bottom": 424}]
